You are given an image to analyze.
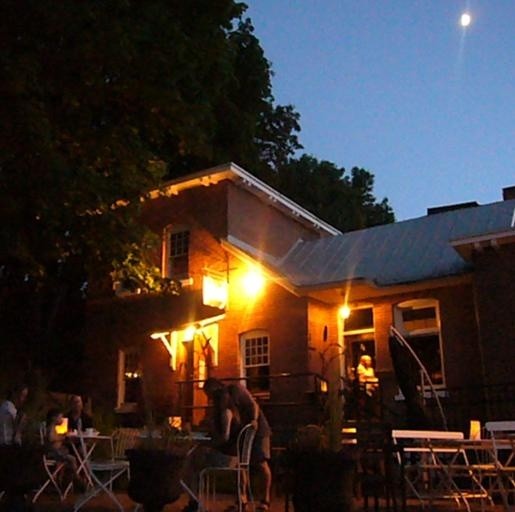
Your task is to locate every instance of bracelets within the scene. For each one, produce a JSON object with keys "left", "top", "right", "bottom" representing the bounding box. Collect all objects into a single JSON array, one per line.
[{"left": 252, "top": 415, "right": 257, "bottom": 420}]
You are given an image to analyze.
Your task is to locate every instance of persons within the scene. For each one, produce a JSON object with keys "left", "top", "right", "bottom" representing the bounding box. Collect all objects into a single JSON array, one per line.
[
  {"left": 0, "top": 381, "right": 31, "bottom": 511},
  {"left": 288, "top": 424, "right": 360, "bottom": 511},
  {"left": 201, "top": 375, "right": 274, "bottom": 511},
  {"left": 62, "top": 393, "right": 93, "bottom": 491},
  {"left": 158, "top": 387, "right": 241, "bottom": 511},
  {"left": 44, "top": 406, "right": 95, "bottom": 493},
  {"left": 356, "top": 354, "right": 380, "bottom": 419}
]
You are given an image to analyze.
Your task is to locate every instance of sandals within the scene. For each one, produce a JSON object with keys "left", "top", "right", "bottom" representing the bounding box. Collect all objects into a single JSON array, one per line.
[
  {"left": 256, "top": 500, "right": 270, "bottom": 512},
  {"left": 224, "top": 500, "right": 250, "bottom": 512}
]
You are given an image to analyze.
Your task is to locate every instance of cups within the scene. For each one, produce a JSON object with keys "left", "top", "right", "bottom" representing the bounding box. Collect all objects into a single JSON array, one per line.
[{"left": 86, "top": 427, "right": 93, "bottom": 437}]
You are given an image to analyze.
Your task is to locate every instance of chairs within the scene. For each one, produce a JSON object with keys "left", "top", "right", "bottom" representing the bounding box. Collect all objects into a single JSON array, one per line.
[
  {"left": 391, "top": 421, "right": 515, "bottom": 512},
  {"left": 32, "top": 421, "right": 256, "bottom": 512}
]
